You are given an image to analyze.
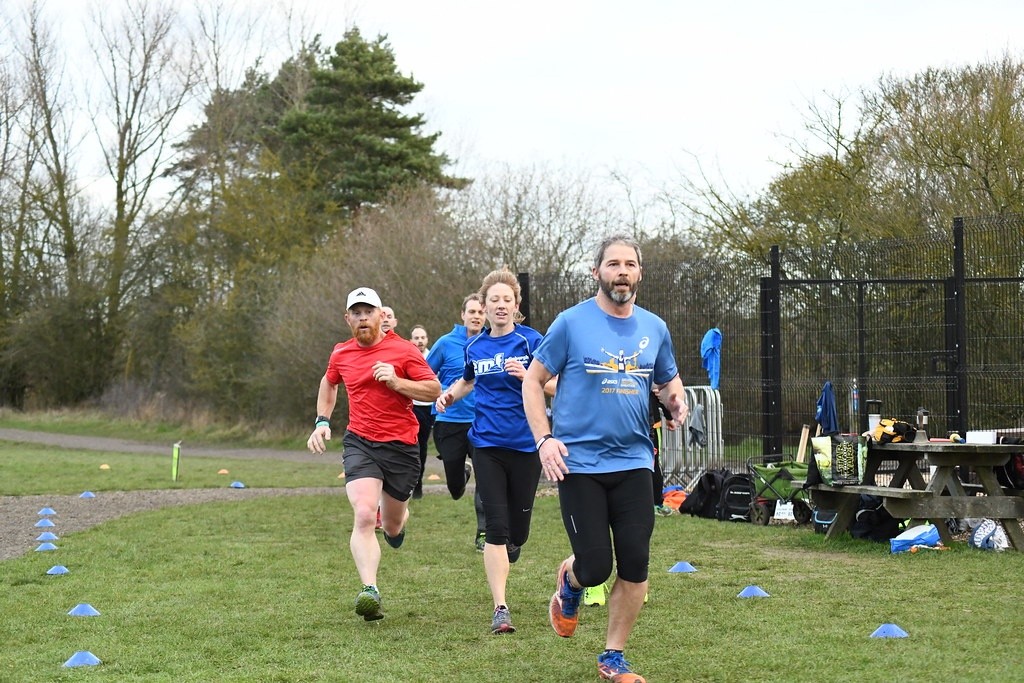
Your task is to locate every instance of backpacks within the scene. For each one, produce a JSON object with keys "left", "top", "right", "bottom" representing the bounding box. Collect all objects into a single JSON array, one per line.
[
  {"left": 715, "top": 472, "right": 757, "bottom": 522},
  {"left": 687, "top": 468, "right": 734, "bottom": 521}
]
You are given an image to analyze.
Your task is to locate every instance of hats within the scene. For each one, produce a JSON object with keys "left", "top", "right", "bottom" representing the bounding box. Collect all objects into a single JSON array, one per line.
[{"left": 346, "top": 286, "right": 383, "bottom": 312}]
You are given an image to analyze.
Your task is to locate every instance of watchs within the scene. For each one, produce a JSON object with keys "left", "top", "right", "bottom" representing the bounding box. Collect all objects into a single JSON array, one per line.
[{"left": 315, "top": 416, "right": 330, "bottom": 424}]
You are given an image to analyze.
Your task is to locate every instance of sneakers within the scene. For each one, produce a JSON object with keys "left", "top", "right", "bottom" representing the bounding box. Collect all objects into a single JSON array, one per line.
[
  {"left": 642, "top": 593, "right": 649, "bottom": 605},
  {"left": 549, "top": 559, "right": 585, "bottom": 638},
  {"left": 596, "top": 655, "right": 647, "bottom": 683},
  {"left": 383, "top": 524, "right": 406, "bottom": 548},
  {"left": 507, "top": 543, "right": 522, "bottom": 564},
  {"left": 490, "top": 604, "right": 516, "bottom": 634},
  {"left": 584, "top": 582, "right": 610, "bottom": 607},
  {"left": 374, "top": 506, "right": 384, "bottom": 531},
  {"left": 464, "top": 461, "right": 473, "bottom": 482},
  {"left": 474, "top": 531, "right": 486, "bottom": 553},
  {"left": 354, "top": 583, "right": 385, "bottom": 620}
]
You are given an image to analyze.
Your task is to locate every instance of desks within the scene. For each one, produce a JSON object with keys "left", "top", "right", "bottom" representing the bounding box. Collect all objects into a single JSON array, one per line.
[{"left": 821, "top": 443, "right": 1024, "bottom": 555}]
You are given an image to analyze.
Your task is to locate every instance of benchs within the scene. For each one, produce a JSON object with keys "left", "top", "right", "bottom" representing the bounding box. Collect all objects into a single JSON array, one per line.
[
  {"left": 944, "top": 483, "right": 1024, "bottom": 497},
  {"left": 790, "top": 480, "right": 934, "bottom": 500}
]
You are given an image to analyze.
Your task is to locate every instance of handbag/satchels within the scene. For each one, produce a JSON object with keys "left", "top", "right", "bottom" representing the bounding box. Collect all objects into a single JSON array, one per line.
[
  {"left": 968, "top": 517, "right": 1013, "bottom": 553},
  {"left": 890, "top": 522, "right": 943, "bottom": 555},
  {"left": 810, "top": 511, "right": 839, "bottom": 536},
  {"left": 849, "top": 504, "right": 899, "bottom": 543}
]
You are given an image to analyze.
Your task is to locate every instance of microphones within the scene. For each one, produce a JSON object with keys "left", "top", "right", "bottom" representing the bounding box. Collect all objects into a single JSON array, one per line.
[{"left": 950, "top": 433, "right": 965, "bottom": 444}]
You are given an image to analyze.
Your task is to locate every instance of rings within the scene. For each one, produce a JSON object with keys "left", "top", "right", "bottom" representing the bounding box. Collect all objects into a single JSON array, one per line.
[{"left": 514, "top": 368, "right": 517, "bottom": 372}]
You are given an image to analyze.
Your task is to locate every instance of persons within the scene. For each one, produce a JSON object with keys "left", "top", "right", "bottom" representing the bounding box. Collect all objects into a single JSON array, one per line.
[
  {"left": 380, "top": 306, "right": 399, "bottom": 334},
  {"left": 436, "top": 269, "right": 560, "bottom": 633},
  {"left": 522, "top": 231, "right": 689, "bottom": 683},
  {"left": 425, "top": 293, "right": 487, "bottom": 554},
  {"left": 307, "top": 287, "right": 442, "bottom": 621},
  {"left": 410, "top": 326, "right": 432, "bottom": 499}
]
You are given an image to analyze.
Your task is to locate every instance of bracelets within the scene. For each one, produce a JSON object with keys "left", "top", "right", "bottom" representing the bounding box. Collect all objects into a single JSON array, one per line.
[
  {"left": 536, "top": 434, "right": 553, "bottom": 450},
  {"left": 316, "top": 421, "right": 330, "bottom": 427}
]
